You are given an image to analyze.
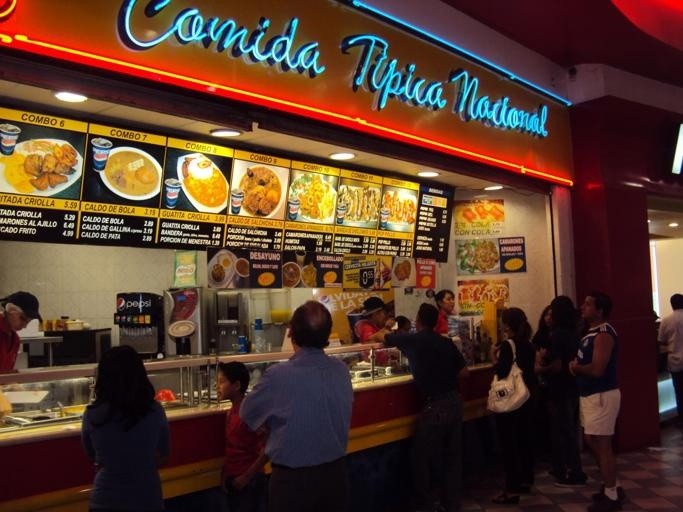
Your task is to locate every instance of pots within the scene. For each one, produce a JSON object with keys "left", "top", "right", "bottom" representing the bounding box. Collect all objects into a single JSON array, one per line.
[{"left": 64, "top": 321, "right": 83, "bottom": 331}]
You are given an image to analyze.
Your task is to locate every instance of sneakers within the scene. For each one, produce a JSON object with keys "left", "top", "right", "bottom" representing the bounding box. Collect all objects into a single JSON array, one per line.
[
  {"left": 588, "top": 487, "right": 625, "bottom": 512},
  {"left": 554, "top": 469, "right": 586, "bottom": 487}
]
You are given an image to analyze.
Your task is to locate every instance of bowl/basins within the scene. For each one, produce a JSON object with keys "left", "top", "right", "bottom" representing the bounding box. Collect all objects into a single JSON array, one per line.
[{"left": 152, "top": 389, "right": 177, "bottom": 400}]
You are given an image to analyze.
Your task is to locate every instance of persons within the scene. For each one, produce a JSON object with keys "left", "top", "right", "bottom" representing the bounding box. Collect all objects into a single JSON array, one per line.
[
  {"left": 216, "top": 360, "right": 267, "bottom": 511},
  {"left": 569, "top": 290, "right": 625, "bottom": 512},
  {"left": 658, "top": 293, "right": 683, "bottom": 432},
  {"left": 79, "top": 344, "right": 171, "bottom": 511},
  {"left": 434, "top": 290, "right": 455, "bottom": 334},
  {"left": 239, "top": 299, "right": 355, "bottom": 510},
  {"left": 0, "top": 291, "right": 43, "bottom": 371},
  {"left": 354, "top": 295, "right": 400, "bottom": 368},
  {"left": 491, "top": 292, "right": 588, "bottom": 494},
  {"left": 376, "top": 303, "right": 470, "bottom": 512}
]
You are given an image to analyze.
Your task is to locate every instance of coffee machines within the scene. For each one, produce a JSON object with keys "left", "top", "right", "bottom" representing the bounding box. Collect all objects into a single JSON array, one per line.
[{"left": 213, "top": 289, "right": 246, "bottom": 354}]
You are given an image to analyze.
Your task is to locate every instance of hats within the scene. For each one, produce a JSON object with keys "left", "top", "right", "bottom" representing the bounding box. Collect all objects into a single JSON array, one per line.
[
  {"left": 0, "top": 291, "right": 43, "bottom": 323},
  {"left": 362, "top": 297, "right": 386, "bottom": 316}
]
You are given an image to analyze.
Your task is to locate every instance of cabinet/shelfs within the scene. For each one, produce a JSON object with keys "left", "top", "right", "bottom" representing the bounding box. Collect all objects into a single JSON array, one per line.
[{"left": 0, "top": 335, "right": 492, "bottom": 512}]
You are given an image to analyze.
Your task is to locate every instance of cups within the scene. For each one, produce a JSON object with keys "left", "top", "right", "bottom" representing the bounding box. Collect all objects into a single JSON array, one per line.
[
  {"left": 344, "top": 311, "right": 364, "bottom": 343},
  {"left": 335, "top": 205, "right": 346, "bottom": 225},
  {"left": 237, "top": 336, "right": 247, "bottom": 354},
  {"left": 254, "top": 317, "right": 265, "bottom": 352},
  {"left": 230, "top": 189, "right": 244, "bottom": 214},
  {"left": 233, "top": 258, "right": 249, "bottom": 278},
  {"left": 295, "top": 250, "right": 306, "bottom": 267},
  {"left": 378, "top": 209, "right": 389, "bottom": 230},
  {"left": 88, "top": 137, "right": 112, "bottom": 172},
  {"left": 0, "top": 123, "right": 21, "bottom": 156},
  {"left": 163, "top": 177, "right": 181, "bottom": 210},
  {"left": 287, "top": 198, "right": 301, "bottom": 219}
]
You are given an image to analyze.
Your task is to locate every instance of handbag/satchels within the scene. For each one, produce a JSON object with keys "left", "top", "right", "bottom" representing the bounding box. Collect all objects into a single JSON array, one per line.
[{"left": 487, "top": 362, "right": 530, "bottom": 413}]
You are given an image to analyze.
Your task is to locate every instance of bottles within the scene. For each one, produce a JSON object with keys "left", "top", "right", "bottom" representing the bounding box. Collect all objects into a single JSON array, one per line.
[
  {"left": 207, "top": 338, "right": 216, "bottom": 354},
  {"left": 474, "top": 325, "right": 493, "bottom": 362}
]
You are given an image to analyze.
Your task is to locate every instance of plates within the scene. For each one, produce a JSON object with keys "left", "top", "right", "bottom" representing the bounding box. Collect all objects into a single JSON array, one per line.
[
  {"left": 240, "top": 164, "right": 283, "bottom": 218},
  {"left": 381, "top": 189, "right": 417, "bottom": 226},
  {"left": 288, "top": 176, "right": 337, "bottom": 223},
  {"left": 0, "top": 138, "right": 82, "bottom": 196},
  {"left": 300, "top": 265, "right": 316, "bottom": 287},
  {"left": 457, "top": 245, "right": 500, "bottom": 273},
  {"left": 282, "top": 262, "right": 301, "bottom": 288},
  {"left": 176, "top": 153, "right": 230, "bottom": 214},
  {"left": 100, "top": 146, "right": 162, "bottom": 202},
  {"left": 207, "top": 249, "right": 236, "bottom": 288},
  {"left": 339, "top": 185, "right": 379, "bottom": 228}
]
